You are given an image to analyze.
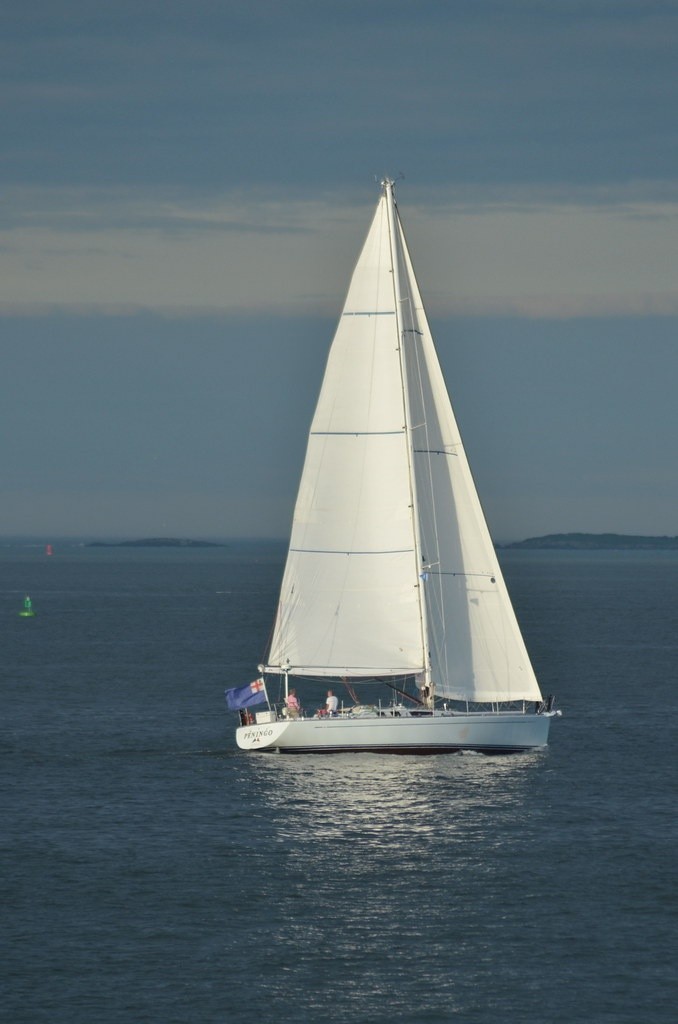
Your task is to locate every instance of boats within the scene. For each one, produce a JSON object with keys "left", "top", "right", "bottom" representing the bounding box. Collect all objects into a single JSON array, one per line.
[
  {"left": 18, "top": 594, "right": 36, "bottom": 618},
  {"left": 46, "top": 543, "right": 54, "bottom": 556}
]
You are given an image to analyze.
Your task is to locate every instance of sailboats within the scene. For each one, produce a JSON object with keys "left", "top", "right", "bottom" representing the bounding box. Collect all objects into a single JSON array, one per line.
[{"left": 231, "top": 167, "right": 566, "bottom": 757}]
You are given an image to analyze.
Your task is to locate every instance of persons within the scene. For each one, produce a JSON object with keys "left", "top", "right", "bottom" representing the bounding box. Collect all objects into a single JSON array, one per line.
[
  {"left": 287, "top": 688, "right": 300, "bottom": 718},
  {"left": 319, "top": 690, "right": 339, "bottom": 717}
]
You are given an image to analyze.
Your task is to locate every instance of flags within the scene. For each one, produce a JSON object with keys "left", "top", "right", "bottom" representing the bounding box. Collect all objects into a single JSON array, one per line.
[{"left": 224, "top": 678, "right": 268, "bottom": 712}]
